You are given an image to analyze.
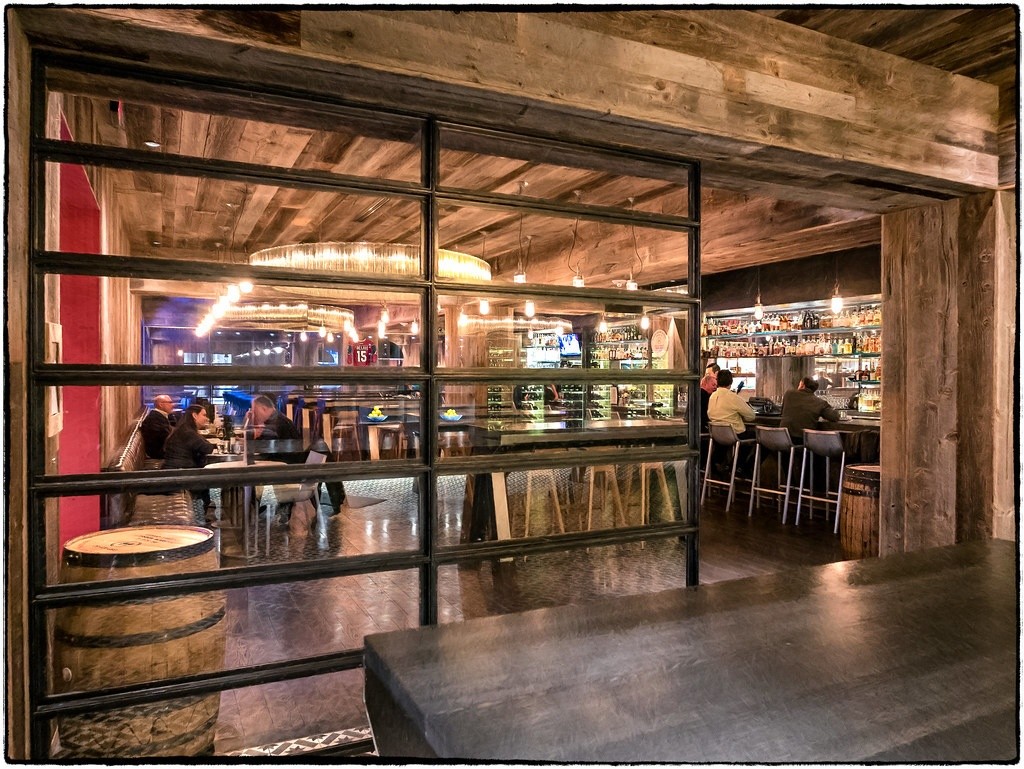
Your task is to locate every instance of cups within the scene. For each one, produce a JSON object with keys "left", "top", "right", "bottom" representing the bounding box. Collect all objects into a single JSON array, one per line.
[{"left": 217, "top": 441, "right": 228, "bottom": 453}]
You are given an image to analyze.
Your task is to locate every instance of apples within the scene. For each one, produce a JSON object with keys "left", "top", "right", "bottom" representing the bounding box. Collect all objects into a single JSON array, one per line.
[{"left": 368, "top": 406, "right": 383, "bottom": 417}]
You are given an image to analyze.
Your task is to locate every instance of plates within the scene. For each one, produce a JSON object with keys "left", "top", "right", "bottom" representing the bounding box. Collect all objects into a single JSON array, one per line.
[
  {"left": 366, "top": 415, "right": 388, "bottom": 422},
  {"left": 440, "top": 414, "right": 463, "bottom": 422}
]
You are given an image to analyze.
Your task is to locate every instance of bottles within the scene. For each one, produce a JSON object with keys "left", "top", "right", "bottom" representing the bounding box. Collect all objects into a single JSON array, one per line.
[
  {"left": 624, "top": 326, "right": 639, "bottom": 340},
  {"left": 856, "top": 358, "right": 882, "bottom": 382},
  {"left": 858, "top": 387, "right": 881, "bottom": 413},
  {"left": 700, "top": 305, "right": 882, "bottom": 355}
]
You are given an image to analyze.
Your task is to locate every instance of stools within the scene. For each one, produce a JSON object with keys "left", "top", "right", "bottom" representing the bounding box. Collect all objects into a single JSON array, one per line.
[
  {"left": 255, "top": 451, "right": 328, "bottom": 557},
  {"left": 223, "top": 391, "right": 253, "bottom": 415},
  {"left": 578, "top": 420, "right": 631, "bottom": 553},
  {"left": 627, "top": 420, "right": 681, "bottom": 550},
  {"left": 796, "top": 428, "right": 857, "bottom": 534},
  {"left": 748, "top": 424, "right": 813, "bottom": 523},
  {"left": 701, "top": 433, "right": 711, "bottom": 497},
  {"left": 701, "top": 421, "right": 760, "bottom": 512},
  {"left": 506, "top": 422, "right": 568, "bottom": 562}
]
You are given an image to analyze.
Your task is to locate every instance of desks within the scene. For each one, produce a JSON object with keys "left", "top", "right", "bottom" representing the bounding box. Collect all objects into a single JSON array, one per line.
[
  {"left": 198, "top": 421, "right": 289, "bottom": 560},
  {"left": 700, "top": 417, "right": 881, "bottom": 514},
  {"left": 276, "top": 391, "right": 687, "bottom": 578},
  {"left": 363, "top": 538, "right": 1016, "bottom": 762}
]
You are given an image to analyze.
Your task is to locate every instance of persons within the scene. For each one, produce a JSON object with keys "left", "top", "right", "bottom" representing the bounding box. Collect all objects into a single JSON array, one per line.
[
  {"left": 142, "top": 394, "right": 174, "bottom": 459},
  {"left": 525, "top": 385, "right": 559, "bottom": 410},
  {"left": 779, "top": 375, "right": 842, "bottom": 499},
  {"left": 512, "top": 385, "right": 526, "bottom": 410},
  {"left": 706, "top": 363, "right": 720, "bottom": 379},
  {"left": 706, "top": 369, "right": 761, "bottom": 483},
  {"left": 163, "top": 404, "right": 216, "bottom": 521},
  {"left": 244, "top": 396, "right": 303, "bottom": 526},
  {"left": 682, "top": 376, "right": 726, "bottom": 498}
]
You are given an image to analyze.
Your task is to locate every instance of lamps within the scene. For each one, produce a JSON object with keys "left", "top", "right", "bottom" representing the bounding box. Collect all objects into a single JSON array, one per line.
[
  {"left": 301, "top": 306, "right": 418, "bottom": 342},
  {"left": 475, "top": 262, "right": 641, "bottom": 319},
  {"left": 196, "top": 303, "right": 357, "bottom": 333},
  {"left": 224, "top": 343, "right": 282, "bottom": 361},
  {"left": 249, "top": 240, "right": 491, "bottom": 302}
]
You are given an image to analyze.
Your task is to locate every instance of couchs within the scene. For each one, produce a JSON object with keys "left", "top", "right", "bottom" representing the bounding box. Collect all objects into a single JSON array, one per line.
[{"left": 106, "top": 403, "right": 197, "bottom": 533}]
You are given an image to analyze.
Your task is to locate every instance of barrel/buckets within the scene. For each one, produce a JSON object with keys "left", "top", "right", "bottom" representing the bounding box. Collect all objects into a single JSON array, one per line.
[
  {"left": 50, "top": 524, "right": 227, "bottom": 760},
  {"left": 839, "top": 463, "right": 880, "bottom": 562}
]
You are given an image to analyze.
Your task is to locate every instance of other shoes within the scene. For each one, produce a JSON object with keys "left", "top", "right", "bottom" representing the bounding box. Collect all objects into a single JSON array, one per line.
[{"left": 728, "top": 472, "right": 750, "bottom": 491}]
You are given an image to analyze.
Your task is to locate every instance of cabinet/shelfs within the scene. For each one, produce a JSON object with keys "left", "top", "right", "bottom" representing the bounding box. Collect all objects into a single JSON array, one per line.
[
  {"left": 700, "top": 301, "right": 881, "bottom": 356},
  {"left": 586, "top": 317, "right": 685, "bottom": 419},
  {"left": 857, "top": 355, "right": 883, "bottom": 414}
]
